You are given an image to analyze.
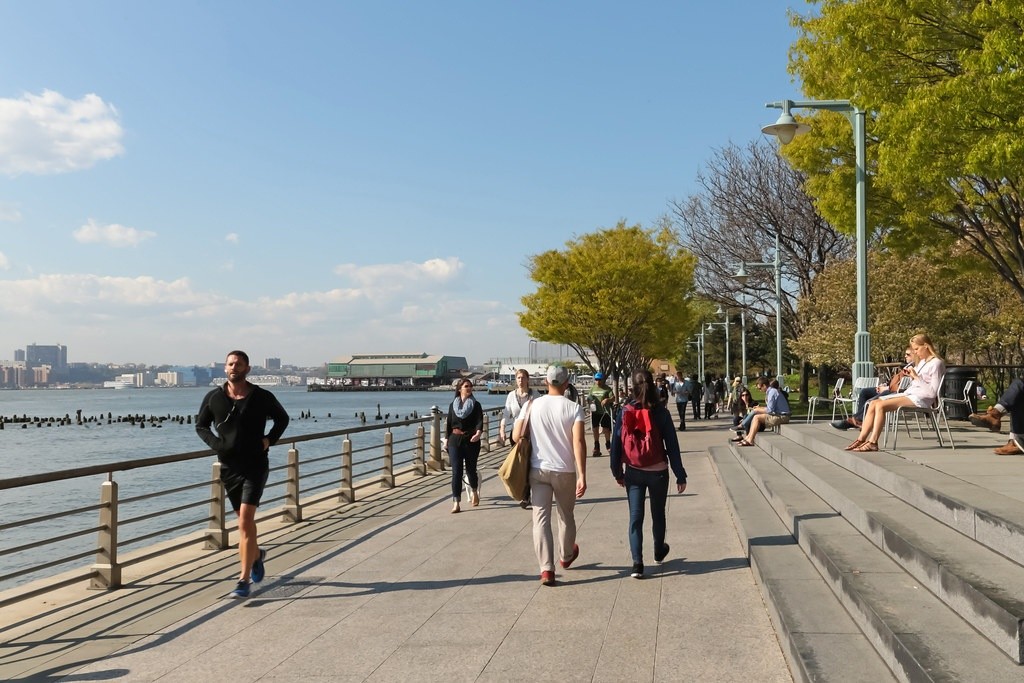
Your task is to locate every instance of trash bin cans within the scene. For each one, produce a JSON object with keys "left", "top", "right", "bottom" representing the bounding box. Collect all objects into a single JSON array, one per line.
[{"left": 944, "top": 365, "right": 979, "bottom": 420}]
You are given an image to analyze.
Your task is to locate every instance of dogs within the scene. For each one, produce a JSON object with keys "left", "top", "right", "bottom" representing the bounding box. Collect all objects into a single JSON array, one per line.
[{"left": 465, "top": 466, "right": 486, "bottom": 503}]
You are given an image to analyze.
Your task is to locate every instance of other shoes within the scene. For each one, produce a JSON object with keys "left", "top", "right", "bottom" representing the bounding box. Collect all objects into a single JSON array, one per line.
[
  {"left": 471, "top": 495, "right": 480, "bottom": 506},
  {"left": 452, "top": 506, "right": 460, "bottom": 512},
  {"left": 694, "top": 415, "right": 701, "bottom": 420},
  {"left": 704, "top": 416, "right": 711, "bottom": 420},
  {"left": 731, "top": 435, "right": 744, "bottom": 442},
  {"left": 679, "top": 423, "right": 686, "bottom": 431}
]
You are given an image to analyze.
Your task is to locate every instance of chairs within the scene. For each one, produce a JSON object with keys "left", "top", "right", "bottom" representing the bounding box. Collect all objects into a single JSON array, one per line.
[{"left": 807, "top": 378, "right": 974, "bottom": 450}]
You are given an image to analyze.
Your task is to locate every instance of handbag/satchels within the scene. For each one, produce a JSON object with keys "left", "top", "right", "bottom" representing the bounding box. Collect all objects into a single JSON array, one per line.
[{"left": 498, "top": 437, "right": 531, "bottom": 500}]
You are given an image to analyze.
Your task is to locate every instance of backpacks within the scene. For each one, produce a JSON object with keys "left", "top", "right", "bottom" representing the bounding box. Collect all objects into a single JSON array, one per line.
[{"left": 621, "top": 403, "right": 661, "bottom": 468}]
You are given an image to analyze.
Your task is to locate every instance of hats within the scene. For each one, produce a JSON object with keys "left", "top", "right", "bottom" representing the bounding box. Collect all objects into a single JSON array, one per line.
[
  {"left": 546, "top": 364, "right": 570, "bottom": 385},
  {"left": 594, "top": 372, "right": 603, "bottom": 380}
]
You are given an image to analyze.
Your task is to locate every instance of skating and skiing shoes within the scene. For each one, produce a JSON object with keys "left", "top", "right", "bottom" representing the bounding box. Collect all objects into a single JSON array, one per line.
[{"left": 593, "top": 448, "right": 611, "bottom": 457}]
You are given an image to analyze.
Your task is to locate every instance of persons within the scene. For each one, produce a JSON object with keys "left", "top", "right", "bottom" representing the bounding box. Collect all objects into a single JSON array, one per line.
[
  {"left": 969, "top": 379, "right": 1024, "bottom": 454},
  {"left": 196, "top": 351, "right": 289, "bottom": 596},
  {"left": 563, "top": 371, "right": 792, "bottom": 456},
  {"left": 499, "top": 369, "right": 540, "bottom": 508},
  {"left": 445, "top": 377, "right": 483, "bottom": 513},
  {"left": 610, "top": 370, "right": 687, "bottom": 577},
  {"left": 830, "top": 333, "right": 946, "bottom": 451},
  {"left": 513, "top": 365, "right": 587, "bottom": 585}
]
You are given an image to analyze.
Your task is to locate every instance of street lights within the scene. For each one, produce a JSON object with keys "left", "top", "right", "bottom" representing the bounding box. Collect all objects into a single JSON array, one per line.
[
  {"left": 684, "top": 333, "right": 702, "bottom": 384},
  {"left": 703, "top": 309, "right": 736, "bottom": 400},
  {"left": 730, "top": 232, "right": 785, "bottom": 389},
  {"left": 761, "top": 100, "right": 875, "bottom": 416},
  {"left": 687, "top": 322, "right": 711, "bottom": 391}
]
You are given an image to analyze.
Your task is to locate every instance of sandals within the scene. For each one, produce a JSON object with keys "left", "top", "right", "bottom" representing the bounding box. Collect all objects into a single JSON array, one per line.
[
  {"left": 844, "top": 439, "right": 865, "bottom": 450},
  {"left": 852, "top": 440, "right": 878, "bottom": 452}
]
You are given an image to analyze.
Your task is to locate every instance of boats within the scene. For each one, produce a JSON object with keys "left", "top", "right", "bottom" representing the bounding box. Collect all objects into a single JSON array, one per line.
[{"left": 486, "top": 373, "right": 632, "bottom": 397}]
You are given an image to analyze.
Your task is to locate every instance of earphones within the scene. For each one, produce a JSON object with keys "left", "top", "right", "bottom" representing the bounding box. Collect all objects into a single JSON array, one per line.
[{"left": 224, "top": 369, "right": 228, "bottom": 374}]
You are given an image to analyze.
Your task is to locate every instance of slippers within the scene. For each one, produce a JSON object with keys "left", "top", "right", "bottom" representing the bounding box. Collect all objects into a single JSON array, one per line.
[
  {"left": 738, "top": 440, "right": 754, "bottom": 446},
  {"left": 737, "top": 438, "right": 746, "bottom": 445}
]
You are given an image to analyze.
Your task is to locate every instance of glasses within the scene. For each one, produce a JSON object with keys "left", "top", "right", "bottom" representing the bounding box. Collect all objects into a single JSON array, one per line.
[
  {"left": 741, "top": 393, "right": 748, "bottom": 396},
  {"left": 462, "top": 385, "right": 473, "bottom": 388}
]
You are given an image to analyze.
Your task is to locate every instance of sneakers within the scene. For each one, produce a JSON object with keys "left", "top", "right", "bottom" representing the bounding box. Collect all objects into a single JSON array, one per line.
[
  {"left": 993, "top": 439, "right": 1024, "bottom": 455},
  {"left": 230, "top": 579, "right": 254, "bottom": 598},
  {"left": 829, "top": 419, "right": 858, "bottom": 432},
  {"left": 560, "top": 543, "right": 579, "bottom": 567},
  {"left": 251, "top": 549, "right": 266, "bottom": 582},
  {"left": 540, "top": 571, "right": 555, "bottom": 585},
  {"left": 654, "top": 543, "right": 669, "bottom": 563},
  {"left": 730, "top": 426, "right": 747, "bottom": 433},
  {"left": 968, "top": 405, "right": 1002, "bottom": 431},
  {"left": 631, "top": 565, "right": 644, "bottom": 578}
]
